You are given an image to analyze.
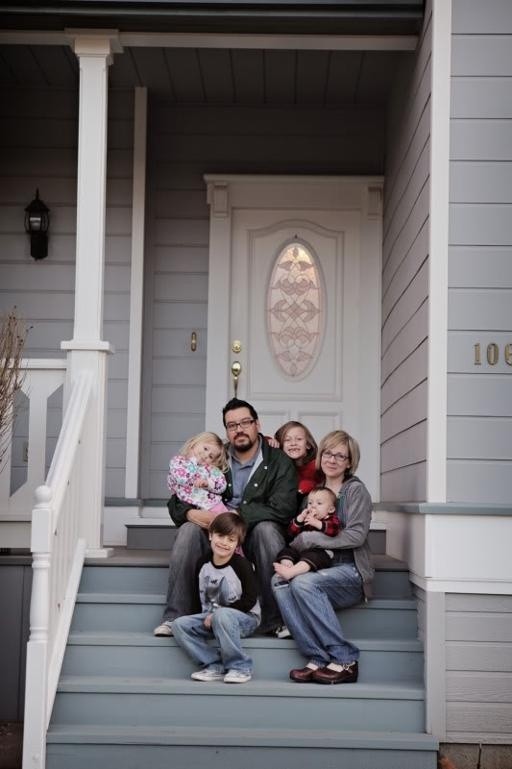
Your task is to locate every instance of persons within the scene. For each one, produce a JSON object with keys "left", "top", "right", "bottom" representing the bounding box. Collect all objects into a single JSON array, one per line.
[
  {"left": 272, "top": 486, "right": 342, "bottom": 580},
  {"left": 153, "top": 399, "right": 297, "bottom": 640},
  {"left": 167, "top": 430, "right": 245, "bottom": 559},
  {"left": 264, "top": 420, "right": 319, "bottom": 494},
  {"left": 172, "top": 512, "right": 263, "bottom": 684},
  {"left": 270, "top": 430, "right": 375, "bottom": 684}
]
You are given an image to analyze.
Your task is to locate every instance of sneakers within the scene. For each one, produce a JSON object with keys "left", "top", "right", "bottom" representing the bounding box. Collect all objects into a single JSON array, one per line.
[
  {"left": 222, "top": 668, "right": 253, "bottom": 683},
  {"left": 191, "top": 664, "right": 226, "bottom": 681},
  {"left": 153, "top": 618, "right": 174, "bottom": 636},
  {"left": 276, "top": 625, "right": 293, "bottom": 639}
]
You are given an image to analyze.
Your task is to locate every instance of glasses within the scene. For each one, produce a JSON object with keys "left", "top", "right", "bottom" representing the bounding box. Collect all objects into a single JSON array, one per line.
[
  {"left": 225, "top": 419, "right": 258, "bottom": 430},
  {"left": 322, "top": 450, "right": 352, "bottom": 461}
]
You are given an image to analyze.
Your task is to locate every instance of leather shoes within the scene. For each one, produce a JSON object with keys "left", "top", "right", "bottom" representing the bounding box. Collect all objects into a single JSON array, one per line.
[
  {"left": 313, "top": 660, "right": 358, "bottom": 685},
  {"left": 289, "top": 659, "right": 322, "bottom": 681}
]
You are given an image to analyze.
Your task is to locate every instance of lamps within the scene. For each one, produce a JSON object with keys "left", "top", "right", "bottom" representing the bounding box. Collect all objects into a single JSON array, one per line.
[{"left": 24, "top": 187, "right": 50, "bottom": 261}]
